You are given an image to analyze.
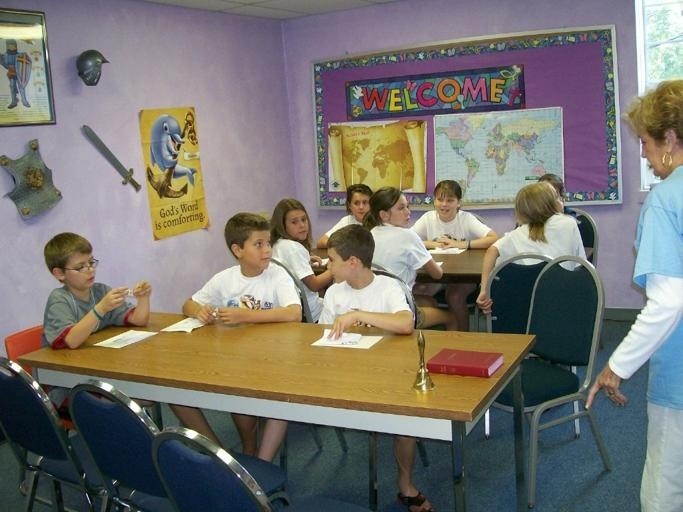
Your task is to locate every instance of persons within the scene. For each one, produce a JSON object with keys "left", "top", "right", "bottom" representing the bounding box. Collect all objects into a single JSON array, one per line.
[
  {"left": 259, "top": 223, "right": 438, "bottom": 512},
  {"left": 317, "top": 184, "right": 375, "bottom": 248},
  {"left": 271, "top": 199, "right": 334, "bottom": 324},
  {"left": 1, "top": 39, "right": 31, "bottom": 109},
  {"left": 41, "top": 234, "right": 153, "bottom": 422},
  {"left": 585, "top": 78, "right": 683, "bottom": 510},
  {"left": 539, "top": 174, "right": 595, "bottom": 264},
  {"left": 182, "top": 213, "right": 301, "bottom": 454},
  {"left": 411, "top": 179, "right": 497, "bottom": 333},
  {"left": 362, "top": 187, "right": 459, "bottom": 331},
  {"left": 476, "top": 183, "right": 587, "bottom": 365}
]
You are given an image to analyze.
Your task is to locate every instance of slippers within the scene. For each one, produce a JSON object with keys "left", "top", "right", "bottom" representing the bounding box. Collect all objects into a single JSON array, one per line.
[{"left": 397, "top": 491, "right": 435, "bottom": 512}]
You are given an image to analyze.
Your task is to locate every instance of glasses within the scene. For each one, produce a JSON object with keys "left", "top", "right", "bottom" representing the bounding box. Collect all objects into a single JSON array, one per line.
[{"left": 66, "top": 258, "right": 100, "bottom": 274}]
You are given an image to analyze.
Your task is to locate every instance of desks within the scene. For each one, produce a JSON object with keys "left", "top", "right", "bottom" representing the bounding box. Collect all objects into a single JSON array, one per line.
[
  {"left": 307, "top": 246, "right": 593, "bottom": 282},
  {"left": 17, "top": 303, "right": 538, "bottom": 512}
]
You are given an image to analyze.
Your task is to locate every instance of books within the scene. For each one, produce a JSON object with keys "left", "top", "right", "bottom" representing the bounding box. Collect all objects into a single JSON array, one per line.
[{"left": 425, "top": 347, "right": 503, "bottom": 377}]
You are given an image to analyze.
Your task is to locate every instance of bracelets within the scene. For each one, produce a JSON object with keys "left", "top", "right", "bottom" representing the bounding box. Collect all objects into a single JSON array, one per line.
[
  {"left": 92, "top": 306, "right": 104, "bottom": 322},
  {"left": 467, "top": 239, "right": 472, "bottom": 248}
]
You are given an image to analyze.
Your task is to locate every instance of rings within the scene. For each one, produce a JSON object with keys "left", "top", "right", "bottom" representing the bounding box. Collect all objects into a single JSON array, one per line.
[{"left": 609, "top": 391, "right": 615, "bottom": 397}]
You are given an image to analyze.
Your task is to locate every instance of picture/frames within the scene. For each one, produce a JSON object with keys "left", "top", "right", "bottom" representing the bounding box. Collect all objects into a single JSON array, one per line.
[{"left": 0, "top": 8, "right": 58, "bottom": 127}]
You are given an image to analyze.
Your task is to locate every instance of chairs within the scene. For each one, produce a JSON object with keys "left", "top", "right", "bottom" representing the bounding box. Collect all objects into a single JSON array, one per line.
[
  {"left": 150, "top": 425, "right": 377, "bottom": 512},
  {"left": 67, "top": 377, "right": 290, "bottom": 509},
  {"left": 483, "top": 255, "right": 613, "bottom": 511},
  {"left": 0, "top": 355, "right": 112, "bottom": 512},
  {"left": 309, "top": 268, "right": 434, "bottom": 511},
  {"left": 217, "top": 258, "right": 345, "bottom": 486},
  {"left": 563, "top": 208, "right": 597, "bottom": 253},
  {"left": 3, "top": 321, "right": 111, "bottom": 510},
  {"left": 472, "top": 213, "right": 486, "bottom": 225},
  {"left": 466, "top": 252, "right": 583, "bottom": 442}
]
